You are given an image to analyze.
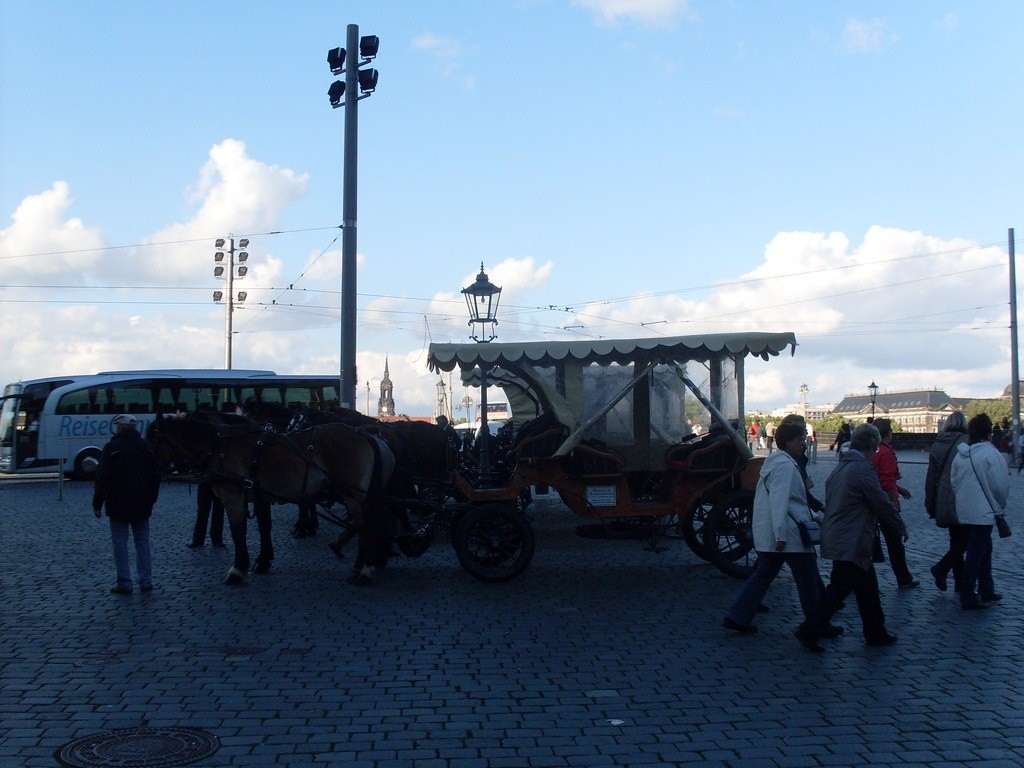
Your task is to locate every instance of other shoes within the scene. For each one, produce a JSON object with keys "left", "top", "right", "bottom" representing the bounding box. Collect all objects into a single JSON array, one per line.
[
  {"left": 929, "top": 564, "right": 948, "bottom": 591},
  {"left": 794, "top": 631, "right": 825, "bottom": 655},
  {"left": 981, "top": 592, "right": 1002, "bottom": 602},
  {"left": 211, "top": 541, "right": 225, "bottom": 548},
  {"left": 110, "top": 585, "right": 132, "bottom": 592},
  {"left": 819, "top": 627, "right": 844, "bottom": 639},
  {"left": 140, "top": 584, "right": 154, "bottom": 591},
  {"left": 187, "top": 540, "right": 202, "bottom": 548},
  {"left": 899, "top": 579, "right": 918, "bottom": 587},
  {"left": 866, "top": 632, "right": 898, "bottom": 646},
  {"left": 721, "top": 616, "right": 759, "bottom": 635}
]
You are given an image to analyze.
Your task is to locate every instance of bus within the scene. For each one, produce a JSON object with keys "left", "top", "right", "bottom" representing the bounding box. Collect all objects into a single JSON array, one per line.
[
  {"left": 1, "top": 371, "right": 341, "bottom": 479},
  {"left": 476, "top": 401, "right": 509, "bottom": 423}
]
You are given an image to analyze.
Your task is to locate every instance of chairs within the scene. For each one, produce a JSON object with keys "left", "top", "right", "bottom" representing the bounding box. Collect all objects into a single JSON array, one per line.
[
  {"left": 138, "top": 404, "right": 149, "bottom": 413},
  {"left": 174, "top": 402, "right": 188, "bottom": 413},
  {"left": 163, "top": 403, "right": 173, "bottom": 413},
  {"left": 116, "top": 403, "right": 126, "bottom": 413},
  {"left": 309, "top": 401, "right": 320, "bottom": 408},
  {"left": 78, "top": 402, "right": 89, "bottom": 413},
  {"left": 94, "top": 404, "right": 101, "bottom": 413},
  {"left": 221, "top": 401, "right": 233, "bottom": 412},
  {"left": 127, "top": 402, "right": 138, "bottom": 413},
  {"left": 152, "top": 402, "right": 163, "bottom": 412},
  {"left": 328, "top": 400, "right": 338, "bottom": 407},
  {"left": 103, "top": 402, "right": 113, "bottom": 413},
  {"left": 288, "top": 401, "right": 298, "bottom": 409}
]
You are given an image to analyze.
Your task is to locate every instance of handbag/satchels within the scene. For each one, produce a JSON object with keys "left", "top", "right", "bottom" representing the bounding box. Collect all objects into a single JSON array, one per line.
[
  {"left": 800, "top": 521, "right": 822, "bottom": 548},
  {"left": 871, "top": 527, "right": 885, "bottom": 563},
  {"left": 994, "top": 514, "right": 1011, "bottom": 538}
]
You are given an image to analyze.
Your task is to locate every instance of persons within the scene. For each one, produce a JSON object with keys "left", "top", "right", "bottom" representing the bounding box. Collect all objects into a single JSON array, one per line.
[
  {"left": 92, "top": 414, "right": 163, "bottom": 595},
  {"left": 187, "top": 400, "right": 321, "bottom": 549},
  {"left": 436, "top": 416, "right": 463, "bottom": 452},
  {"left": 721, "top": 412, "right": 1024, "bottom": 652},
  {"left": 25, "top": 414, "right": 39, "bottom": 432}
]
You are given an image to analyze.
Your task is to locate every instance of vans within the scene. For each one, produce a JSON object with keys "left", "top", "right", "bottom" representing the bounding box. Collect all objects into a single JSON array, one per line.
[{"left": 453, "top": 421, "right": 506, "bottom": 451}]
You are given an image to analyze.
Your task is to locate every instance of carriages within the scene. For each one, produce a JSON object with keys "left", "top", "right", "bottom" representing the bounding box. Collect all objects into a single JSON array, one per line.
[{"left": 157, "top": 333, "right": 798, "bottom": 586}]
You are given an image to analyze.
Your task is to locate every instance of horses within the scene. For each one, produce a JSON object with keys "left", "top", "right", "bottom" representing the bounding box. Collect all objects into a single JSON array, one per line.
[{"left": 146, "top": 399, "right": 449, "bottom": 590}]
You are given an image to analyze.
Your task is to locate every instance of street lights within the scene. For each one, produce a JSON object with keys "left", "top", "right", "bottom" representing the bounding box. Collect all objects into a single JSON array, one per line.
[
  {"left": 461, "top": 260, "right": 501, "bottom": 471},
  {"left": 868, "top": 381, "right": 879, "bottom": 418},
  {"left": 799, "top": 383, "right": 810, "bottom": 419},
  {"left": 327, "top": 34, "right": 381, "bottom": 412},
  {"left": 436, "top": 380, "right": 446, "bottom": 416}
]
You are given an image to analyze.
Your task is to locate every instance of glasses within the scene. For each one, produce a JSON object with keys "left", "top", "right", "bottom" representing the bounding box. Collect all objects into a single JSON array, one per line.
[{"left": 872, "top": 447, "right": 880, "bottom": 453}]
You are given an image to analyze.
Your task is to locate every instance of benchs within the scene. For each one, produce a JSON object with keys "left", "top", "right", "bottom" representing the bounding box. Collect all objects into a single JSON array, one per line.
[
  {"left": 666, "top": 418, "right": 747, "bottom": 472},
  {"left": 571, "top": 439, "right": 626, "bottom": 474},
  {"left": 515, "top": 423, "right": 567, "bottom": 458}
]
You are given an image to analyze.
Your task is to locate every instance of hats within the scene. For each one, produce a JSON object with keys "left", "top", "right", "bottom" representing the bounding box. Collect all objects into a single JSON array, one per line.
[{"left": 112, "top": 414, "right": 137, "bottom": 425}]
